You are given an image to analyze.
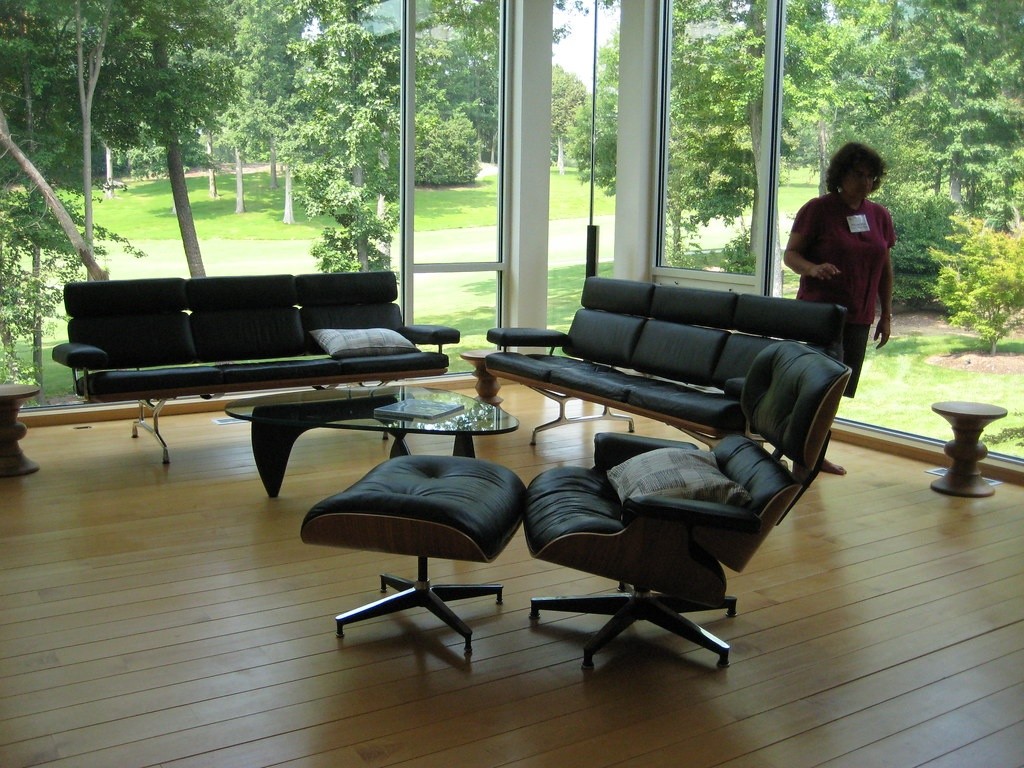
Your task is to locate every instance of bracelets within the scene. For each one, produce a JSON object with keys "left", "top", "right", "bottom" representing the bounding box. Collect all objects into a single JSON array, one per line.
[{"left": 881, "top": 314, "right": 890, "bottom": 321}]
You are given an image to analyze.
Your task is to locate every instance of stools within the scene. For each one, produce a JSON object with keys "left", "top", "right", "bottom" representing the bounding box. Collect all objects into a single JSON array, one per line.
[
  {"left": 931, "top": 400, "right": 1008, "bottom": 498},
  {"left": 0, "top": 384, "right": 40, "bottom": 478},
  {"left": 460, "top": 349, "right": 504, "bottom": 412},
  {"left": 300, "top": 454, "right": 528, "bottom": 657}
]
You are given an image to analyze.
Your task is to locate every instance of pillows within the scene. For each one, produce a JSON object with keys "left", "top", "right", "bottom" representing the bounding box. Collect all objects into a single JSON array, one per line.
[
  {"left": 605, "top": 447, "right": 753, "bottom": 521},
  {"left": 309, "top": 328, "right": 421, "bottom": 360}
]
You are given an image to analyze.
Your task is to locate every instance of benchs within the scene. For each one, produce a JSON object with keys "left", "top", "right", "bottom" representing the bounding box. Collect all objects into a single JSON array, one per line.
[
  {"left": 484, "top": 276, "right": 849, "bottom": 467},
  {"left": 52, "top": 272, "right": 461, "bottom": 464}
]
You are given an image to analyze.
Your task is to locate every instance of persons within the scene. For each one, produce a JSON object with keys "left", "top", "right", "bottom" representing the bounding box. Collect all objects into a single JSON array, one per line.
[{"left": 783, "top": 142, "right": 897, "bottom": 475}]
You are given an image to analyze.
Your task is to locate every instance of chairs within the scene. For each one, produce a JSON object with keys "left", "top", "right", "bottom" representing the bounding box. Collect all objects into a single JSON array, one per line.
[{"left": 521, "top": 338, "right": 853, "bottom": 671}]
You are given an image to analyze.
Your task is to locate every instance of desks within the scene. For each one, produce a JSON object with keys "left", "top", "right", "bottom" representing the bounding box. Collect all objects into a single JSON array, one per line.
[{"left": 223, "top": 386, "right": 521, "bottom": 498}]
